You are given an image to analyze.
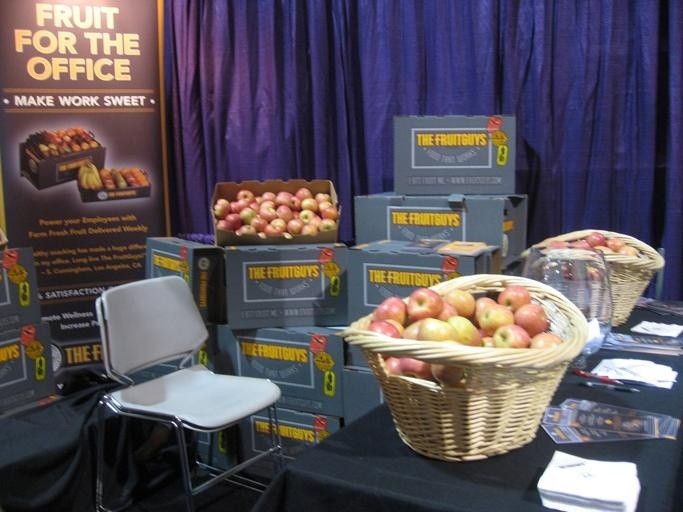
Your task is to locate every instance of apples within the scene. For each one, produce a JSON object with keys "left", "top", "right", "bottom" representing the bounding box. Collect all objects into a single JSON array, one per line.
[
  {"left": 400, "top": 357, "right": 432, "bottom": 379},
  {"left": 478, "top": 329, "right": 491, "bottom": 337},
  {"left": 260, "top": 201, "right": 276, "bottom": 208},
  {"left": 235, "top": 229, "right": 239, "bottom": 236},
  {"left": 374, "top": 297, "right": 407, "bottom": 326},
  {"left": 435, "top": 297, "right": 458, "bottom": 321},
  {"left": 493, "top": 324, "right": 531, "bottom": 348},
  {"left": 250, "top": 198, "right": 260, "bottom": 214},
  {"left": 239, "top": 208, "right": 256, "bottom": 225},
  {"left": 549, "top": 241, "right": 570, "bottom": 249},
  {"left": 514, "top": 304, "right": 550, "bottom": 338},
  {"left": 251, "top": 217, "right": 269, "bottom": 232},
  {"left": 275, "top": 192, "right": 294, "bottom": 208},
  {"left": 586, "top": 232, "right": 606, "bottom": 248},
  {"left": 430, "top": 363, "right": 464, "bottom": 384},
  {"left": 618, "top": 245, "right": 639, "bottom": 257},
  {"left": 255, "top": 197, "right": 262, "bottom": 204},
  {"left": 282, "top": 232, "right": 292, "bottom": 238},
  {"left": 318, "top": 201, "right": 333, "bottom": 212},
  {"left": 217, "top": 198, "right": 230, "bottom": 219},
  {"left": 570, "top": 240, "right": 596, "bottom": 251},
  {"left": 530, "top": 331, "right": 563, "bottom": 348},
  {"left": 214, "top": 203, "right": 226, "bottom": 217},
  {"left": 264, "top": 224, "right": 283, "bottom": 237},
  {"left": 593, "top": 245, "right": 616, "bottom": 254},
  {"left": 301, "top": 224, "right": 317, "bottom": 235},
  {"left": 319, "top": 218, "right": 336, "bottom": 231},
  {"left": 260, "top": 192, "right": 276, "bottom": 203},
  {"left": 309, "top": 214, "right": 322, "bottom": 231},
  {"left": 287, "top": 219, "right": 305, "bottom": 234},
  {"left": 237, "top": 190, "right": 254, "bottom": 202},
  {"left": 230, "top": 202, "right": 239, "bottom": 213},
  {"left": 239, "top": 225, "right": 256, "bottom": 235},
  {"left": 315, "top": 193, "right": 331, "bottom": 204},
  {"left": 407, "top": 288, "right": 444, "bottom": 322},
  {"left": 447, "top": 315, "right": 483, "bottom": 346},
  {"left": 402, "top": 320, "right": 421, "bottom": 338},
  {"left": 478, "top": 304, "right": 515, "bottom": 337},
  {"left": 217, "top": 220, "right": 234, "bottom": 232},
  {"left": 368, "top": 320, "right": 401, "bottom": 339},
  {"left": 385, "top": 356, "right": 401, "bottom": 375},
  {"left": 498, "top": 285, "right": 530, "bottom": 313},
  {"left": 606, "top": 238, "right": 625, "bottom": 252},
  {"left": 301, "top": 198, "right": 318, "bottom": 212},
  {"left": 445, "top": 288, "right": 476, "bottom": 318},
  {"left": 299, "top": 209, "right": 314, "bottom": 225},
  {"left": 321, "top": 206, "right": 338, "bottom": 221},
  {"left": 384, "top": 319, "right": 404, "bottom": 335},
  {"left": 418, "top": 318, "right": 460, "bottom": 342},
  {"left": 257, "top": 231, "right": 266, "bottom": 238},
  {"left": 276, "top": 205, "right": 293, "bottom": 222},
  {"left": 288, "top": 197, "right": 301, "bottom": 211},
  {"left": 473, "top": 297, "right": 497, "bottom": 326},
  {"left": 270, "top": 218, "right": 286, "bottom": 232},
  {"left": 482, "top": 336, "right": 494, "bottom": 347},
  {"left": 292, "top": 211, "right": 299, "bottom": 219},
  {"left": 296, "top": 187, "right": 312, "bottom": 201},
  {"left": 235, "top": 199, "right": 250, "bottom": 214},
  {"left": 259, "top": 206, "right": 277, "bottom": 222},
  {"left": 225, "top": 213, "right": 242, "bottom": 230}
]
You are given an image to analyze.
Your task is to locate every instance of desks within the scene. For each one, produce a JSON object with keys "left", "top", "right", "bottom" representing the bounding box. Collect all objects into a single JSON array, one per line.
[{"left": 285, "top": 301, "right": 682, "bottom": 512}]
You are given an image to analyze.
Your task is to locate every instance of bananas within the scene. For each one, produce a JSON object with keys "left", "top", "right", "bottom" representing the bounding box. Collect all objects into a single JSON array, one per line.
[{"left": 78, "top": 159, "right": 102, "bottom": 191}]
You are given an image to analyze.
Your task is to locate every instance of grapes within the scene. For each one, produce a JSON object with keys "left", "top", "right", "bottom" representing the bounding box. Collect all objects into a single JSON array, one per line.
[{"left": 26, "top": 129, "right": 58, "bottom": 147}]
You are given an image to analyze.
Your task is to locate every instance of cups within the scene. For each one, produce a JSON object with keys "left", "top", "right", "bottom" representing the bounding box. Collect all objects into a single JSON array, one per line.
[{"left": 520, "top": 246, "right": 614, "bottom": 356}]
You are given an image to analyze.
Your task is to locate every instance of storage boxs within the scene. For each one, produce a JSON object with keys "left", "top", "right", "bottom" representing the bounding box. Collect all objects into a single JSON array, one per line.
[
  {"left": 226, "top": 243, "right": 347, "bottom": 329},
  {"left": 348, "top": 240, "right": 501, "bottom": 328},
  {"left": 186, "top": 426, "right": 239, "bottom": 470},
  {"left": 342, "top": 368, "right": 386, "bottom": 423},
  {"left": 239, "top": 408, "right": 343, "bottom": 480},
  {"left": 354, "top": 193, "right": 527, "bottom": 271},
  {"left": 216, "top": 323, "right": 345, "bottom": 419},
  {"left": 0, "top": 322, "right": 57, "bottom": 416},
  {"left": 211, "top": 178, "right": 341, "bottom": 246},
  {"left": 0, "top": 247, "right": 40, "bottom": 333},
  {"left": 144, "top": 236, "right": 226, "bottom": 324},
  {"left": 392, "top": 114, "right": 516, "bottom": 196}
]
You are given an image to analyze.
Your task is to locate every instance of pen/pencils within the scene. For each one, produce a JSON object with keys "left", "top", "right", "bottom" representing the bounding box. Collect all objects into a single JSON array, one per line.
[
  {"left": 574, "top": 368, "right": 624, "bottom": 385},
  {"left": 579, "top": 381, "right": 640, "bottom": 392}
]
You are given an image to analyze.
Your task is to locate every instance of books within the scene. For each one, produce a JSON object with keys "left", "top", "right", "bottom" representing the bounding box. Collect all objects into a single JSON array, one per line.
[{"left": 441, "top": 238, "right": 488, "bottom": 255}]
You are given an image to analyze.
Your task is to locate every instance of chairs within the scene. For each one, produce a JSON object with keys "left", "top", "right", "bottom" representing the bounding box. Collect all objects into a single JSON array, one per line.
[{"left": 95, "top": 276, "right": 284, "bottom": 512}]
